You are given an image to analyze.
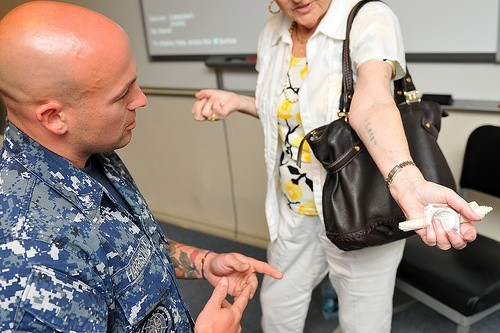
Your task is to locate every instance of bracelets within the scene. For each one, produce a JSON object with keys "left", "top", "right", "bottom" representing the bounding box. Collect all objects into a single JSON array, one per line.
[
  {"left": 201, "top": 251, "right": 214, "bottom": 280},
  {"left": 384, "top": 160, "right": 418, "bottom": 189}
]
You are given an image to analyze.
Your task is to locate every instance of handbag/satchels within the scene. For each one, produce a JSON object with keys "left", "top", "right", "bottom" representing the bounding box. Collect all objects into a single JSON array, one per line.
[{"left": 296, "top": 0, "right": 457, "bottom": 252}]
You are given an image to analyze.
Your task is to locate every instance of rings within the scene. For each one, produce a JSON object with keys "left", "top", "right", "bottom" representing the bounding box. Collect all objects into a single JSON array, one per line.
[{"left": 207, "top": 111, "right": 216, "bottom": 122}]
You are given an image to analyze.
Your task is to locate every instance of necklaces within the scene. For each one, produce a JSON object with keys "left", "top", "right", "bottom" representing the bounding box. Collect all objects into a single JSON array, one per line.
[
  {"left": 282, "top": 20, "right": 302, "bottom": 104},
  {"left": 294, "top": 12, "right": 328, "bottom": 44}
]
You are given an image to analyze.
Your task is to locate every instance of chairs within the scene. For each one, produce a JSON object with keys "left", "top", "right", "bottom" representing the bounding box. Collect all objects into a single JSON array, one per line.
[{"left": 393, "top": 125, "right": 500, "bottom": 333}]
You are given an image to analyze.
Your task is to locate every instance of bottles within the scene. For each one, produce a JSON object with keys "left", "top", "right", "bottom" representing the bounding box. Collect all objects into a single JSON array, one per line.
[{"left": 321, "top": 273, "right": 338, "bottom": 321}]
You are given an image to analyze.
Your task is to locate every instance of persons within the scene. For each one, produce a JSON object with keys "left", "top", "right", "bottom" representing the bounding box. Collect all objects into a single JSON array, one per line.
[
  {"left": 0, "top": 0, "right": 284, "bottom": 333},
  {"left": 190, "top": 0, "right": 482, "bottom": 332}
]
